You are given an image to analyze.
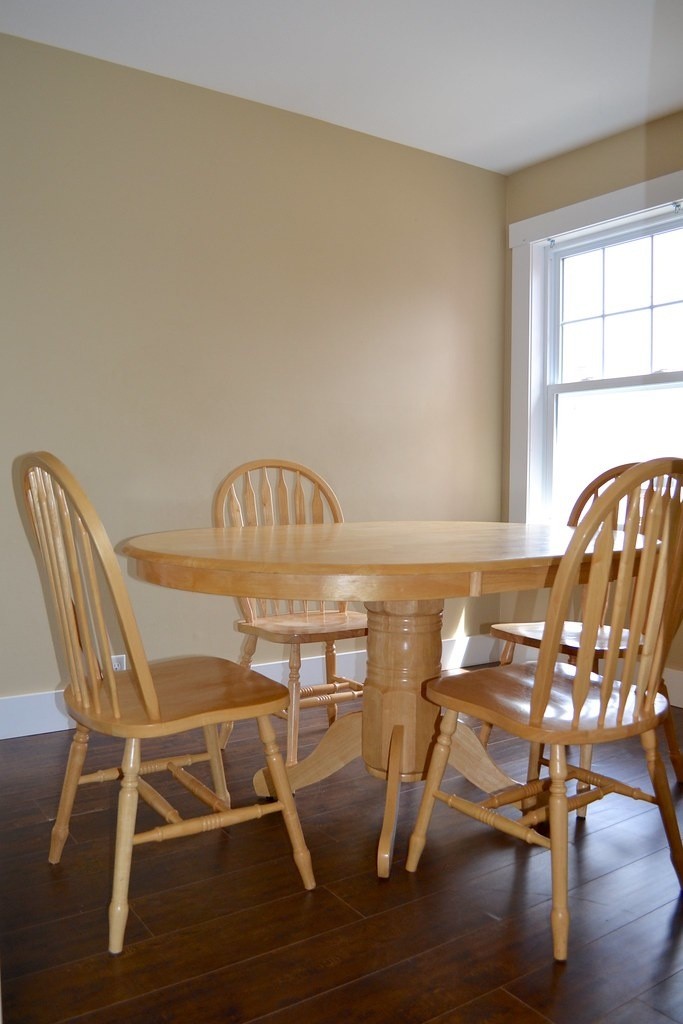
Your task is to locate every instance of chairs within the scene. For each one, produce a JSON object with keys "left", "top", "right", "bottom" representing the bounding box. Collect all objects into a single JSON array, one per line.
[
  {"left": 404, "top": 457, "right": 683, "bottom": 961},
  {"left": 8, "top": 450, "right": 317, "bottom": 957},
  {"left": 481, "top": 460, "right": 683, "bottom": 786},
  {"left": 205, "top": 464, "right": 371, "bottom": 771}
]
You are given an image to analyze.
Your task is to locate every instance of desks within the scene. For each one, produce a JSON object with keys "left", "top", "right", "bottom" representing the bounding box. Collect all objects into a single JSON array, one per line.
[{"left": 114, "top": 511, "right": 672, "bottom": 881}]
